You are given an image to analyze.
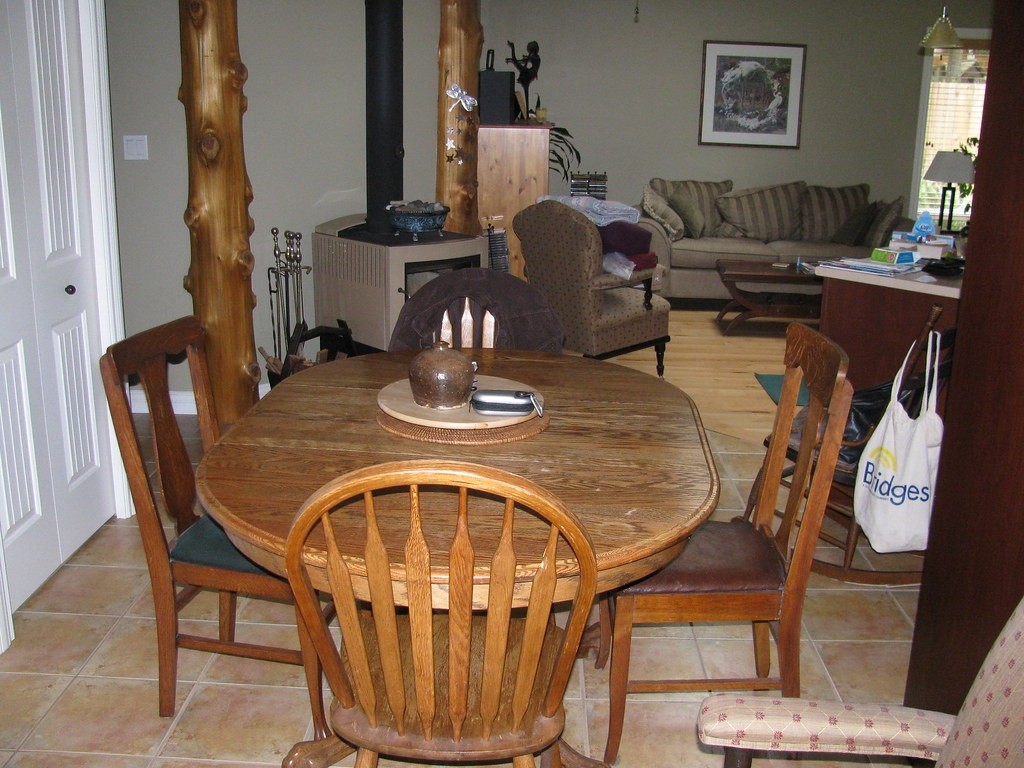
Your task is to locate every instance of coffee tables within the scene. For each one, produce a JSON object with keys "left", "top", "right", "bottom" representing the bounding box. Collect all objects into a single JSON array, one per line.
[{"left": 716, "top": 257, "right": 824, "bottom": 336}]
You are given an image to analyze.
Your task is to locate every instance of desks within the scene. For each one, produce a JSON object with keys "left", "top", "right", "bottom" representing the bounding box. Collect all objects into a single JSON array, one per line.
[{"left": 189, "top": 347, "right": 723, "bottom": 768}]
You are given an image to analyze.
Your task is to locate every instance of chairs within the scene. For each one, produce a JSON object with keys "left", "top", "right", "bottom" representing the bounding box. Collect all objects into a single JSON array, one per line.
[
  {"left": 279, "top": 457, "right": 607, "bottom": 768},
  {"left": 593, "top": 320, "right": 855, "bottom": 768},
  {"left": 387, "top": 265, "right": 566, "bottom": 351},
  {"left": 730, "top": 303, "right": 958, "bottom": 587},
  {"left": 510, "top": 199, "right": 672, "bottom": 381},
  {"left": 98, "top": 312, "right": 338, "bottom": 741},
  {"left": 697, "top": 594, "right": 1024, "bottom": 768}
]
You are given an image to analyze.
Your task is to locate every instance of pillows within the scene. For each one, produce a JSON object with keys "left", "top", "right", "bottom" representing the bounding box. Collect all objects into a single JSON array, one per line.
[
  {"left": 642, "top": 183, "right": 685, "bottom": 242},
  {"left": 715, "top": 180, "right": 806, "bottom": 244},
  {"left": 596, "top": 219, "right": 652, "bottom": 254},
  {"left": 829, "top": 200, "right": 878, "bottom": 246},
  {"left": 801, "top": 183, "right": 871, "bottom": 242},
  {"left": 649, "top": 176, "right": 733, "bottom": 239},
  {"left": 859, "top": 195, "right": 905, "bottom": 247}
]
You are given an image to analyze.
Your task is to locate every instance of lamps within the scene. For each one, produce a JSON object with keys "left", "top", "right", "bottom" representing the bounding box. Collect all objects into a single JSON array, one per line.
[
  {"left": 918, "top": 0, "right": 963, "bottom": 50},
  {"left": 922, "top": 151, "right": 975, "bottom": 236}
]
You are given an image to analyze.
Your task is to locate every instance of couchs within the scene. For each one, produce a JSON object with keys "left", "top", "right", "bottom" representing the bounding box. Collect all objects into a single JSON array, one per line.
[{"left": 633, "top": 204, "right": 917, "bottom": 312}]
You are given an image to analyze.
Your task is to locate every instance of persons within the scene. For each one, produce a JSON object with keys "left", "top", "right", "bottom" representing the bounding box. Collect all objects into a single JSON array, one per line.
[{"left": 505, "top": 39, "right": 541, "bottom": 120}]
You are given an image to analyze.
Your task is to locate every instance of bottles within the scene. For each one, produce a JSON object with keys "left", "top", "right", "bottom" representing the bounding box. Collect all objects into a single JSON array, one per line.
[{"left": 408, "top": 341, "right": 475, "bottom": 410}]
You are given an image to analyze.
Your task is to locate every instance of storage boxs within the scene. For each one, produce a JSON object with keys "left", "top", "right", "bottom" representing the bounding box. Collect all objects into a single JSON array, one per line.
[
  {"left": 871, "top": 247, "right": 915, "bottom": 265},
  {"left": 478, "top": 70, "right": 515, "bottom": 126},
  {"left": 916, "top": 243, "right": 951, "bottom": 260},
  {"left": 889, "top": 239, "right": 918, "bottom": 252}
]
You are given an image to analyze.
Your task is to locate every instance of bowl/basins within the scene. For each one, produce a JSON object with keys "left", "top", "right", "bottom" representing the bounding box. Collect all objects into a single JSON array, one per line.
[{"left": 383, "top": 204, "right": 450, "bottom": 232}]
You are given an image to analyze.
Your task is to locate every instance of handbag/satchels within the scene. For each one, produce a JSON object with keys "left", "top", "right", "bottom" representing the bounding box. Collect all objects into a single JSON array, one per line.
[
  {"left": 853, "top": 329, "right": 944, "bottom": 554},
  {"left": 816, "top": 380, "right": 912, "bottom": 463}
]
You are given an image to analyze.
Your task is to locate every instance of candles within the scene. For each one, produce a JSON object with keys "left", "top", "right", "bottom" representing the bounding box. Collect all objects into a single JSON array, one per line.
[{"left": 536, "top": 108, "right": 548, "bottom": 121}]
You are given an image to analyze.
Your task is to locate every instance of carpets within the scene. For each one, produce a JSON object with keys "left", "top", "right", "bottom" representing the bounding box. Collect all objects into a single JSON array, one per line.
[{"left": 753, "top": 372, "right": 811, "bottom": 407}]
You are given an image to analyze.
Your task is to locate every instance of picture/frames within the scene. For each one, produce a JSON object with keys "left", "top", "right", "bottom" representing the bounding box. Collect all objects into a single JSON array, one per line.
[{"left": 697, "top": 40, "right": 809, "bottom": 151}]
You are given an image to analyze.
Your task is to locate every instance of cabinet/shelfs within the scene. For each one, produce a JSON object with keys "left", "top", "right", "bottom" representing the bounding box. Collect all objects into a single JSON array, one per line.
[
  {"left": 815, "top": 265, "right": 963, "bottom": 391},
  {"left": 571, "top": 170, "right": 607, "bottom": 199},
  {"left": 484, "top": 223, "right": 510, "bottom": 273},
  {"left": 476, "top": 124, "right": 553, "bottom": 284}
]
